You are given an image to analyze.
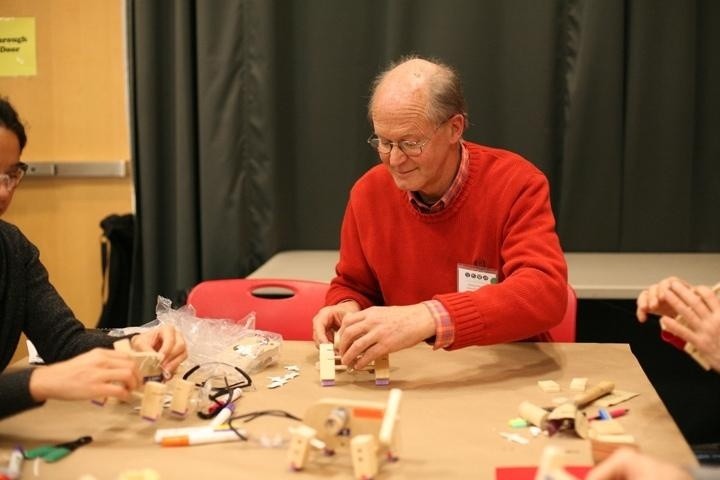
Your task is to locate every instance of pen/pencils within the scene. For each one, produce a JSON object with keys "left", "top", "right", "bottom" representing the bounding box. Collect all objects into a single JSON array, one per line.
[
  {"left": 154, "top": 387, "right": 249, "bottom": 447},
  {"left": 509, "top": 381, "right": 629, "bottom": 437},
  {"left": 7, "top": 446, "right": 24, "bottom": 480}
]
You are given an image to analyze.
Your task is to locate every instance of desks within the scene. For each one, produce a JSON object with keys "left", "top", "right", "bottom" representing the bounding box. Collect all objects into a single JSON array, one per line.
[
  {"left": 0, "top": 337, "right": 706, "bottom": 480},
  {"left": 242, "top": 248, "right": 719, "bottom": 302}
]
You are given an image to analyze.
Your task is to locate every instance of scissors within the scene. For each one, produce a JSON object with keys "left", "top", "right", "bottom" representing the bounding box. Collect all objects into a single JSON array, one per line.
[{"left": 24, "top": 435, "right": 92, "bottom": 462}]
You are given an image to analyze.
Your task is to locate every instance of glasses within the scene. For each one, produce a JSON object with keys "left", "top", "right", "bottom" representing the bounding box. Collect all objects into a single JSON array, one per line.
[
  {"left": 0, "top": 161, "right": 29, "bottom": 188},
  {"left": 366, "top": 114, "right": 453, "bottom": 160}
]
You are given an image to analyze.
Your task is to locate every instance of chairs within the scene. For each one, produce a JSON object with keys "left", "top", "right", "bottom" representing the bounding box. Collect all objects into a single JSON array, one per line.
[{"left": 185, "top": 278, "right": 334, "bottom": 341}]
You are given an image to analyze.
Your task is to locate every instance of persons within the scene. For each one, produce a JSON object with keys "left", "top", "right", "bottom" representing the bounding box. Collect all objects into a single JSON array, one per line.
[
  {"left": 633, "top": 275, "right": 720, "bottom": 375},
  {"left": 0, "top": 96, "right": 188, "bottom": 420},
  {"left": 307, "top": 52, "right": 570, "bottom": 374}
]
